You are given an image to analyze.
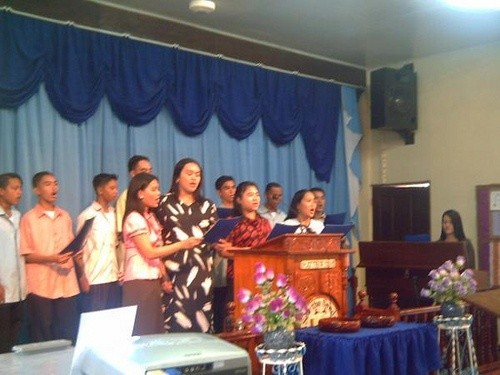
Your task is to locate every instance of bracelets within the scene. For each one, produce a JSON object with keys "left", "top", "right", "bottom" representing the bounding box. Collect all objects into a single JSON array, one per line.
[{"left": 159, "top": 273, "right": 169, "bottom": 284}]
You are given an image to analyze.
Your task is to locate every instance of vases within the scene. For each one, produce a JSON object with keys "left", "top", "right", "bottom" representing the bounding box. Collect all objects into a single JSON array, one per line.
[
  {"left": 263, "top": 328, "right": 295, "bottom": 362},
  {"left": 441, "top": 299, "right": 465, "bottom": 326}
]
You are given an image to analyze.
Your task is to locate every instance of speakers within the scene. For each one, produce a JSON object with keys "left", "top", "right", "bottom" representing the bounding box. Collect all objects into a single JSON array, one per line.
[{"left": 370, "top": 67, "right": 418, "bottom": 131}]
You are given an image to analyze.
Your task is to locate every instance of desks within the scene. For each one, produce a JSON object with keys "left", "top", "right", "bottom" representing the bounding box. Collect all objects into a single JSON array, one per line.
[{"left": 295, "top": 321, "right": 443, "bottom": 375}]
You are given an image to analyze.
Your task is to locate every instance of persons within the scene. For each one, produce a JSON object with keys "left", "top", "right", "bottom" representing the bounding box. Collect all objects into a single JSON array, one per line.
[
  {"left": 0, "top": 156, "right": 345, "bottom": 354},
  {"left": 439, "top": 208, "right": 477, "bottom": 269}
]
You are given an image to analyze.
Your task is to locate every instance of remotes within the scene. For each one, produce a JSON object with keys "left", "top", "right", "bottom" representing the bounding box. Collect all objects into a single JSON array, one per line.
[{"left": 14, "top": 340, "right": 71, "bottom": 354}]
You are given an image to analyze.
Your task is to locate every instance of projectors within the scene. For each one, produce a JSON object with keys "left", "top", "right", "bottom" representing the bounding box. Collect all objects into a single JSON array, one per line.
[{"left": 83, "top": 331, "right": 252, "bottom": 375}]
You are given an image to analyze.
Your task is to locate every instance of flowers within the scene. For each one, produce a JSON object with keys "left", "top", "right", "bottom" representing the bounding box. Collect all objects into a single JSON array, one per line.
[
  {"left": 420, "top": 256, "right": 478, "bottom": 302},
  {"left": 236, "top": 263, "right": 308, "bottom": 335}
]
